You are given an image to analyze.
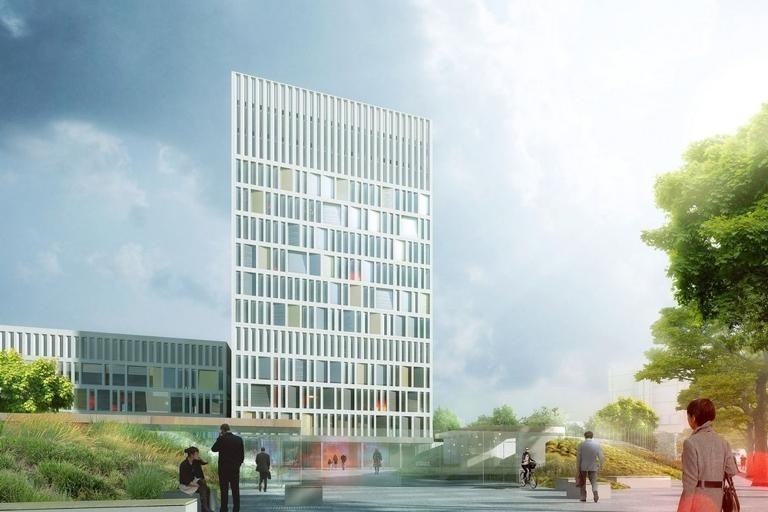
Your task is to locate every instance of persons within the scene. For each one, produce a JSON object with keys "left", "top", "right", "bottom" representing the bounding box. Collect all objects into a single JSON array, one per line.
[
  {"left": 373, "top": 448, "right": 384, "bottom": 473},
  {"left": 328, "top": 458, "right": 333, "bottom": 464},
  {"left": 576, "top": 431, "right": 604, "bottom": 503},
  {"left": 210, "top": 424, "right": 245, "bottom": 512},
  {"left": 179, "top": 446, "right": 214, "bottom": 512},
  {"left": 255, "top": 447, "right": 271, "bottom": 493},
  {"left": 521, "top": 447, "right": 535, "bottom": 481},
  {"left": 333, "top": 454, "right": 339, "bottom": 464},
  {"left": 674, "top": 397, "right": 739, "bottom": 512},
  {"left": 341, "top": 455, "right": 346, "bottom": 471}
]
